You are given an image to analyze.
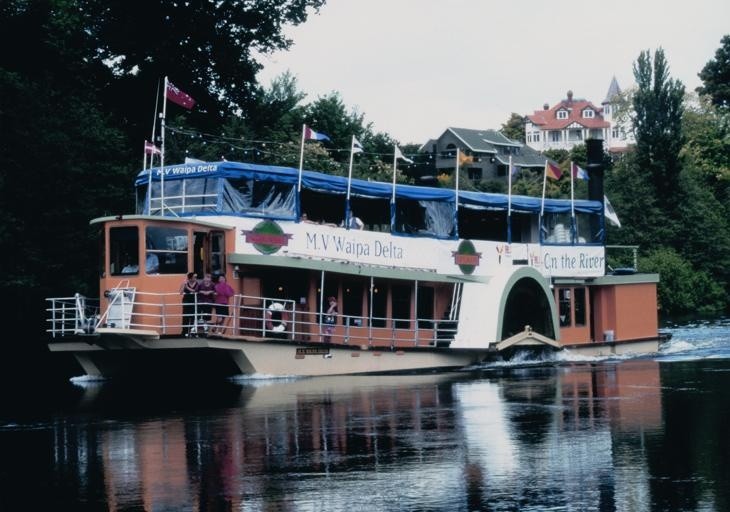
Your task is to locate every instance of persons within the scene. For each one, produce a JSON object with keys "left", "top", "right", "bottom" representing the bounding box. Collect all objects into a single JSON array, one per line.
[
  {"left": 212, "top": 274, "right": 233, "bottom": 335},
  {"left": 325, "top": 296, "right": 337, "bottom": 324},
  {"left": 179, "top": 272, "right": 198, "bottom": 336},
  {"left": 190, "top": 274, "right": 215, "bottom": 333},
  {"left": 144, "top": 246, "right": 159, "bottom": 274},
  {"left": 300, "top": 212, "right": 318, "bottom": 225},
  {"left": 340, "top": 210, "right": 365, "bottom": 230}
]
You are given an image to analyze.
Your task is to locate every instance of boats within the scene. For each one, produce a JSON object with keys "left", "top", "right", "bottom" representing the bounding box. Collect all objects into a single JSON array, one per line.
[{"left": 45, "top": 137, "right": 672, "bottom": 375}]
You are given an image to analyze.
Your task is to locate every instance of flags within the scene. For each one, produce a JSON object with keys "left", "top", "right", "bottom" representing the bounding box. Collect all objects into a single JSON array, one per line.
[
  {"left": 167, "top": 83, "right": 196, "bottom": 110},
  {"left": 573, "top": 164, "right": 590, "bottom": 179},
  {"left": 459, "top": 152, "right": 474, "bottom": 166},
  {"left": 604, "top": 195, "right": 621, "bottom": 228},
  {"left": 396, "top": 145, "right": 414, "bottom": 164},
  {"left": 146, "top": 141, "right": 161, "bottom": 155},
  {"left": 353, "top": 137, "right": 368, "bottom": 157},
  {"left": 546, "top": 162, "right": 562, "bottom": 180},
  {"left": 305, "top": 128, "right": 331, "bottom": 142},
  {"left": 511, "top": 166, "right": 518, "bottom": 181}
]
